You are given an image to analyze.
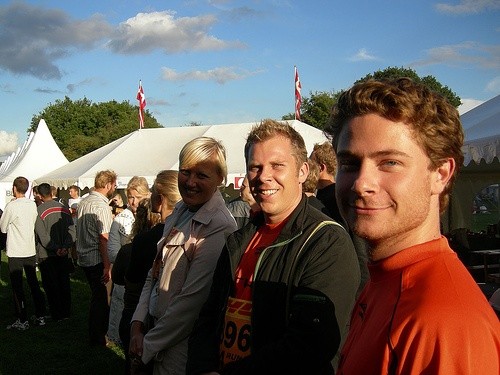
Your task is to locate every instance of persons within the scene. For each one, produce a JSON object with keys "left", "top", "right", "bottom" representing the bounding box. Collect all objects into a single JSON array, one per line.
[
  {"left": 107, "top": 170, "right": 184, "bottom": 375},
  {"left": 334, "top": 77, "right": 500, "bottom": 375},
  {"left": 299, "top": 142, "right": 348, "bottom": 228},
  {"left": 446, "top": 223, "right": 500, "bottom": 313},
  {"left": 184, "top": 118, "right": 362, "bottom": 375},
  {"left": 128, "top": 136, "right": 240, "bottom": 375},
  {"left": 0, "top": 169, "right": 265, "bottom": 348},
  {"left": 486, "top": 187, "right": 496, "bottom": 196}
]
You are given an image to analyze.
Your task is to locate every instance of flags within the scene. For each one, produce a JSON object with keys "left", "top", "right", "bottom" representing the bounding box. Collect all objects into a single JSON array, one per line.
[
  {"left": 295, "top": 66, "right": 303, "bottom": 122},
  {"left": 137, "top": 81, "right": 147, "bottom": 129}
]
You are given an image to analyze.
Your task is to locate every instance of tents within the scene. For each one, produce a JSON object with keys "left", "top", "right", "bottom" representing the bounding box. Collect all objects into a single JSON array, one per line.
[
  {"left": 439, "top": 94, "right": 500, "bottom": 234},
  {"left": 1, "top": 118, "right": 334, "bottom": 215}
]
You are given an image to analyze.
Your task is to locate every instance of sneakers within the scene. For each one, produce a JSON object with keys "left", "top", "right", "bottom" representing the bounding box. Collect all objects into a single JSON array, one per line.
[
  {"left": 33, "top": 317, "right": 46, "bottom": 327},
  {"left": 45, "top": 315, "right": 62, "bottom": 322},
  {"left": 5, "top": 317, "right": 29, "bottom": 331}
]
